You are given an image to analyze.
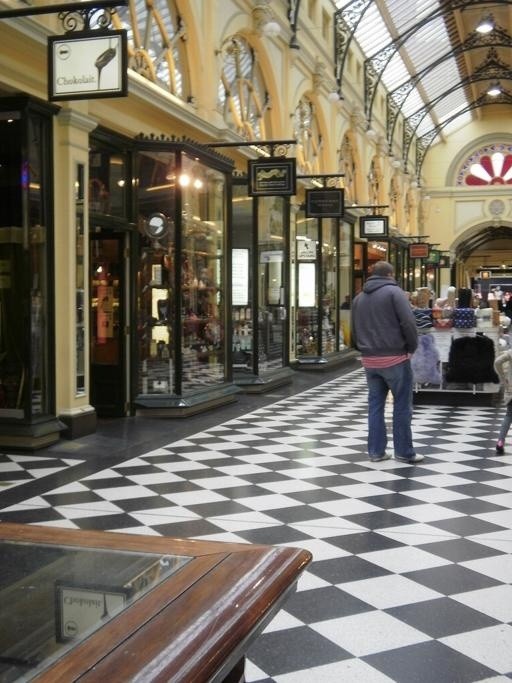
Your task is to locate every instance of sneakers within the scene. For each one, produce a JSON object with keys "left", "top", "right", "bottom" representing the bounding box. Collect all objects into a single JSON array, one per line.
[
  {"left": 496, "top": 444, "right": 505, "bottom": 455},
  {"left": 371, "top": 451, "right": 393, "bottom": 462},
  {"left": 395, "top": 453, "right": 425, "bottom": 463}
]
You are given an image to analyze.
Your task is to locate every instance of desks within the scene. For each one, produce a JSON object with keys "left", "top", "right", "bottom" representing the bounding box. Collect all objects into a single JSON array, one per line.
[{"left": 0, "top": 521, "right": 313, "bottom": 683}]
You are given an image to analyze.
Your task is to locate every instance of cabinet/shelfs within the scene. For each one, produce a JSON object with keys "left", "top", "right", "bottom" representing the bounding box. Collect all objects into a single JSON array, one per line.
[
  {"left": 368, "top": 235, "right": 441, "bottom": 297},
  {"left": 232, "top": 171, "right": 295, "bottom": 394},
  {"left": 289, "top": 202, "right": 358, "bottom": 374},
  {"left": 0, "top": 91, "right": 61, "bottom": 452},
  {"left": 128, "top": 134, "right": 236, "bottom": 423}
]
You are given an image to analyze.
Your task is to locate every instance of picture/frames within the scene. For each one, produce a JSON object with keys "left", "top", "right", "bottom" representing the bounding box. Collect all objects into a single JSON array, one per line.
[
  {"left": 441, "top": 256, "right": 450, "bottom": 268},
  {"left": 47, "top": 29, "right": 129, "bottom": 101},
  {"left": 247, "top": 158, "right": 297, "bottom": 196},
  {"left": 360, "top": 215, "right": 388, "bottom": 238},
  {"left": 305, "top": 188, "right": 345, "bottom": 217}
]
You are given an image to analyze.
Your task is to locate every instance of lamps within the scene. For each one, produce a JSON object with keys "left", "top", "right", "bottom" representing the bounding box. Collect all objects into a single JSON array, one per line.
[
  {"left": 485, "top": 78, "right": 504, "bottom": 96},
  {"left": 476, "top": 7, "right": 496, "bottom": 33},
  {"left": 249, "top": 6, "right": 281, "bottom": 38},
  {"left": 318, "top": 71, "right": 430, "bottom": 204}
]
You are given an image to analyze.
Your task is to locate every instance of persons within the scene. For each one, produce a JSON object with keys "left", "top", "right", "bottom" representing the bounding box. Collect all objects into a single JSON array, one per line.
[
  {"left": 347, "top": 260, "right": 427, "bottom": 465},
  {"left": 499, "top": 290, "right": 511, "bottom": 319},
  {"left": 340, "top": 293, "right": 350, "bottom": 308}
]
